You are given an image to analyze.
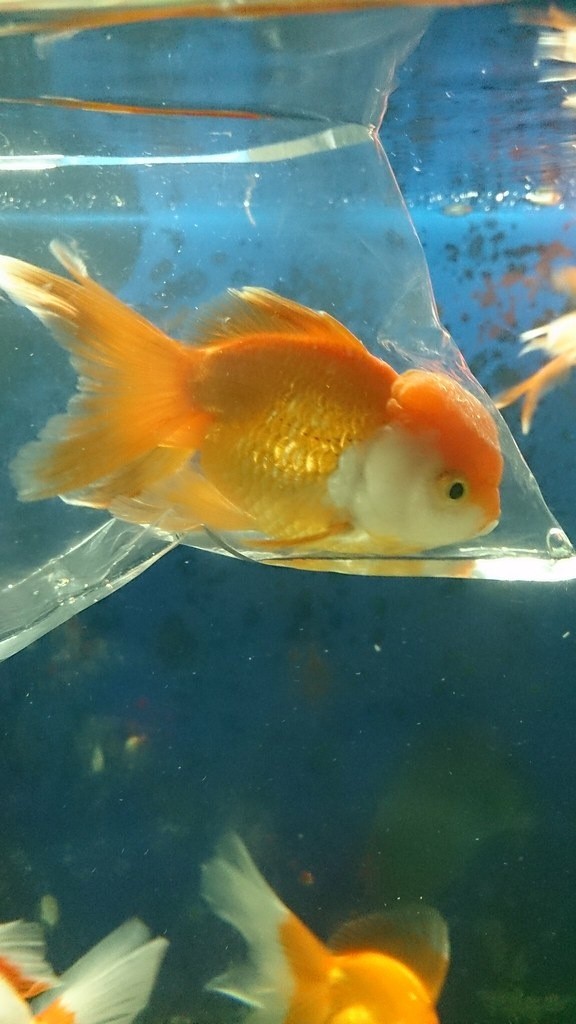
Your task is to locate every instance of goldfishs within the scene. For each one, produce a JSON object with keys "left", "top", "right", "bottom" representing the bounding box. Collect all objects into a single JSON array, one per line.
[
  {"left": 0, "top": 835, "right": 458, "bottom": 1023},
  {"left": 0, "top": 234, "right": 503, "bottom": 563}
]
